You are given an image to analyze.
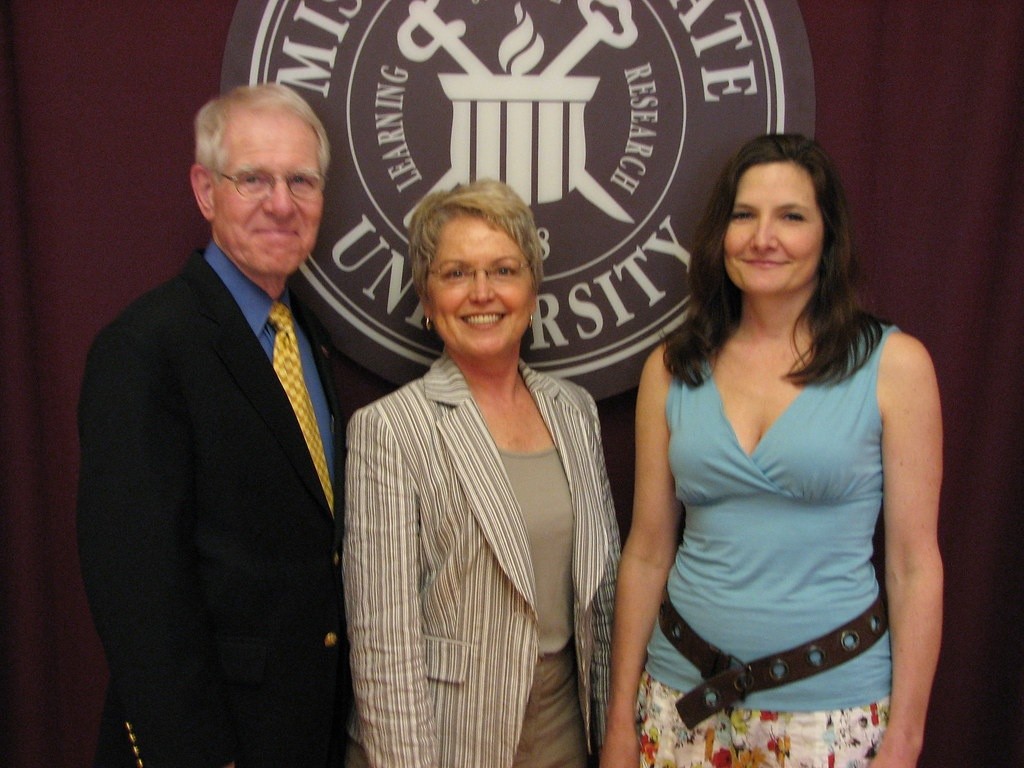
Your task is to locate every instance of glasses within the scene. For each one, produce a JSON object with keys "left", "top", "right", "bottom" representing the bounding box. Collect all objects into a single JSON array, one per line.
[
  {"left": 426, "top": 258, "right": 531, "bottom": 285},
  {"left": 211, "top": 169, "right": 324, "bottom": 201}
]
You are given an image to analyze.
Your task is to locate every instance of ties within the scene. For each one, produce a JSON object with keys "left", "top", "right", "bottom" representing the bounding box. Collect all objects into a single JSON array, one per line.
[{"left": 266, "top": 300, "right": 333, "bottom": 517}]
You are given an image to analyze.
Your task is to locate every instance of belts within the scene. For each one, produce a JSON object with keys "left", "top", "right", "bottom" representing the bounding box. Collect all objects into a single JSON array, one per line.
[{"left": 658, "top": 582, "right": 887, "bottom": 730}]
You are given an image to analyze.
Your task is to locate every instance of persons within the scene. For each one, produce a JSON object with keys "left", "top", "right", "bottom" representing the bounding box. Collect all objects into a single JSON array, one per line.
[
  {"left": 341, "top": 177, "right": 623, "bottom": 768},
  {"left": 76, "top": 83, "right": 356, "bottom": 768},
  {"left": 599, "top": 133, "right": 945, "bottom": 768}
]
